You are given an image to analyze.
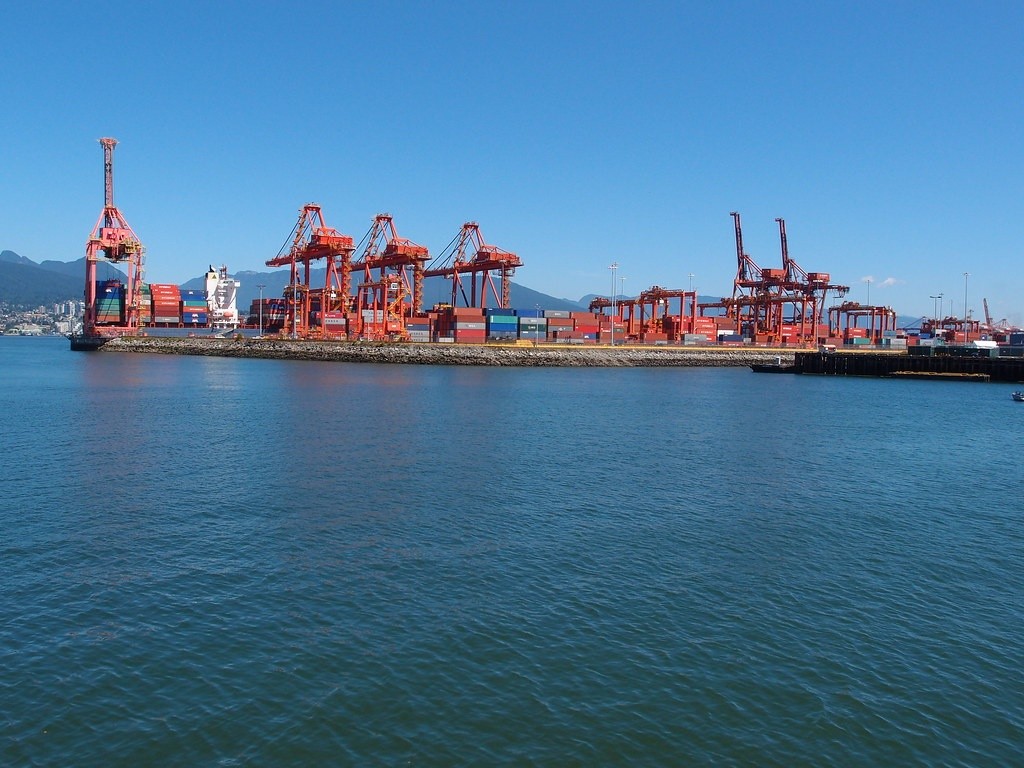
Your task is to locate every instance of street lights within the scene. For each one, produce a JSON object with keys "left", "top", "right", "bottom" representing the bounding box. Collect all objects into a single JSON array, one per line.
[
  {"left": 688, "top": 273, "right": 695, "bottom": 290},
  {"left": 865, "top": 279, "right": 871, "bottom": 329},
  {"left": 931, "top": 295, "right": 942, "bottom": 338},
  {"left": 607, "top": 262, "right": 627, "bottom": 346},
  {"left": 534, "top": 304, "right": 541, "bottom": 346},
  {"left": 256, "top": 285, "right": 266, "bottom": 336},
  {"left": 963, "top": 272, "right": 972, "bottom": 343}
]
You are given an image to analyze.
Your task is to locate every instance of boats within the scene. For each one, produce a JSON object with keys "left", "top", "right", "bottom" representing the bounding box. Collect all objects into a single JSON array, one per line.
[{"left": 1012, "top": 391, "right": 1024, "bottom": 401}]
